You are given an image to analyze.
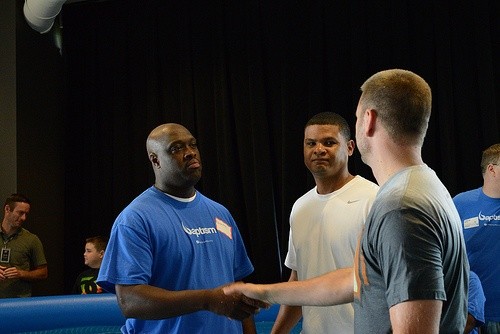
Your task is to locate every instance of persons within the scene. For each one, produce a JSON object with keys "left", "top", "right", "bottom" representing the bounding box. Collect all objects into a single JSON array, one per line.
[
  {"left": 453, "top": 144, "right": 500, "bottom": 334},
  {"left": 0, "top": 194, "right": 48, "bottom": 298},
  {"left": 95, "top": 123, "right": 270, "bottom": 334},
  {"left": 71, "top": 235, "right": 109, "bottom": 295},
  {"left": 271, "top": 112, "right": 379, "bottom": 334},
  {"left": 222, "top": 69, "right": 470, "bottom": 334}
]
